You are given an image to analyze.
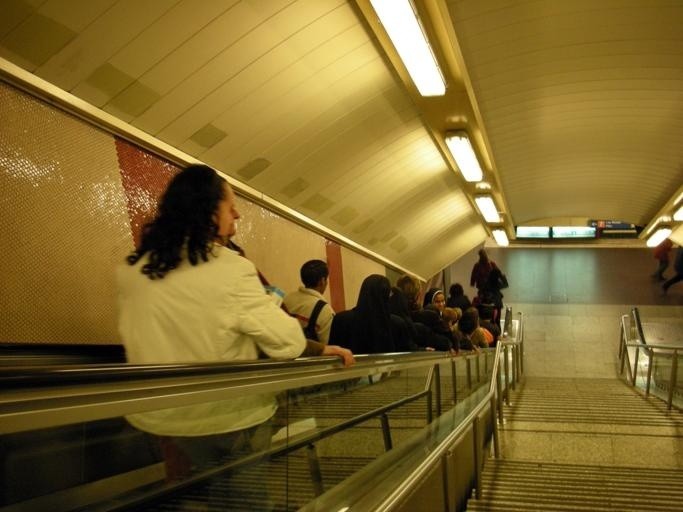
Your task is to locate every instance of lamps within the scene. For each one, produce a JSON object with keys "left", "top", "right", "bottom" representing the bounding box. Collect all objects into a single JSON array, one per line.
[
  {"left": 364, "top": 0, "right": 510, "bottom": 248},
  {"left": 645, "top": 225, "right": 673, "bottom": 249}
]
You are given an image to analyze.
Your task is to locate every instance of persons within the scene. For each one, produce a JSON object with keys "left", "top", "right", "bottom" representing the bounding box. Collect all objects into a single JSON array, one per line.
[
  {"left": 391, "top": 269, "right": 510, "bottom": 352},
  {"left": 278, "top": 260, "right": 335, "bottom": 345},
  {"left": 662, "top": 246, "right": 683, "bottom": 293},
  {"left": 226, "top": 207, "right": 356, "bottom": 368},
  {"left": 118, "top": 165, "right": 308, "bottom": 512},
  {"left": 328, "top": 274, "right": 398, "bottom": 354},
  {"left": 471, "top": 249, "right": 501, "bottom": 288},
  {"left": 651, "top": 238, "right": 673, "bottom": 281}
]
